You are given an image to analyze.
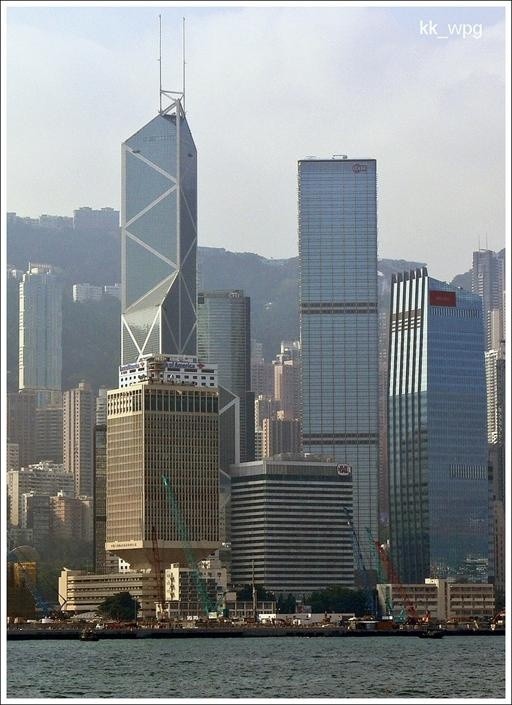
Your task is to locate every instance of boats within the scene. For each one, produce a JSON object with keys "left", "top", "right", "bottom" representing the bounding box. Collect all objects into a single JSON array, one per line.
[
  {"left": 421, "top": 623, "right": 446, "bottom": 639},
  {"left": 81, "top": 628, "right": 98, "bottom": 641}
]
